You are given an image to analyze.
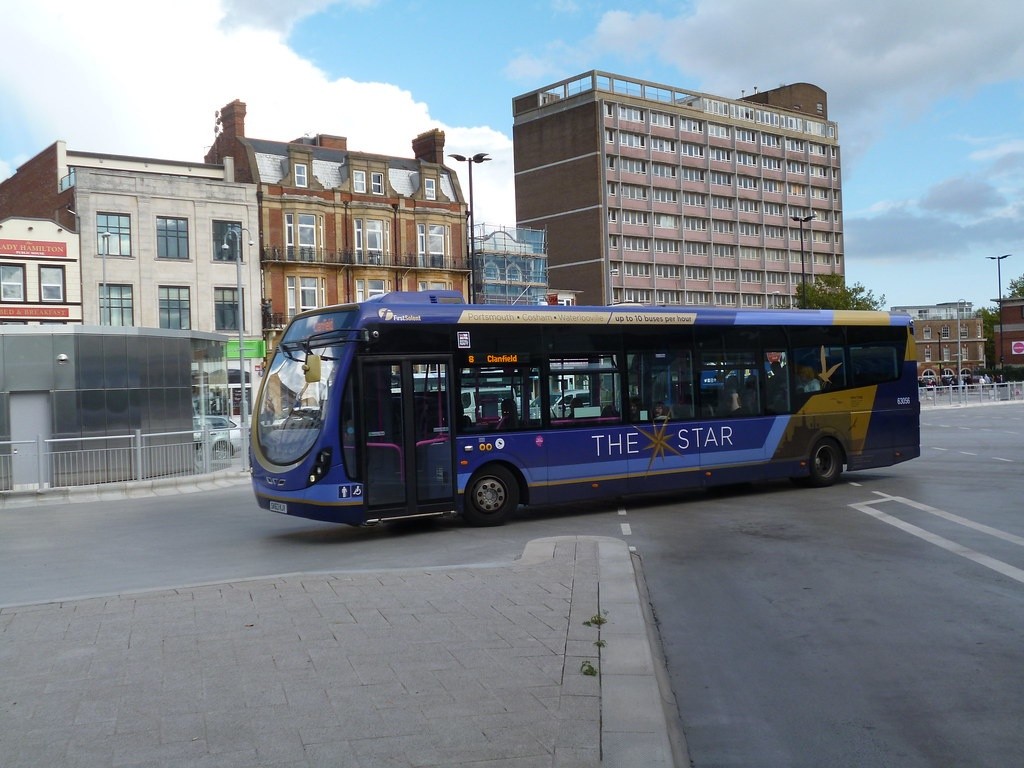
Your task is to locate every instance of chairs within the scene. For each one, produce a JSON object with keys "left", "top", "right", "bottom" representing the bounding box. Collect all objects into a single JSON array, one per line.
[
  {"left": 496, "top": 397, "right": 619, "bottom": 430},
  {"left": 676, "top": 374, "right": 758, "bottom": 418}
]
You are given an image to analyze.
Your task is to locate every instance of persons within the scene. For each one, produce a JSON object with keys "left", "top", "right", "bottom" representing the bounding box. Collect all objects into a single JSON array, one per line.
[
  {"left": 601, "top": 405, "right": 615, "bottom": 416},
  {"left": 629, "top": 398, "right": 641, "bottom": 417},
  {"left": 568, "top": 399, "right": 583, "bottom": 418},
  {"left": 725, "top": 361, "right": 821, "bottom": 414},
  {"left": 500, "top": 399, "right": 517, "bottom": 419},
  {"left": 653, "top": 401, "right": 669, "bottom": 418}
]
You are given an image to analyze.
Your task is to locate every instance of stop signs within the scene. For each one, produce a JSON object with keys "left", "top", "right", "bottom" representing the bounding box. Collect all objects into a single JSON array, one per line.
[{"left": 1012, "top": 341, "right": 1024, "bottom": 354}]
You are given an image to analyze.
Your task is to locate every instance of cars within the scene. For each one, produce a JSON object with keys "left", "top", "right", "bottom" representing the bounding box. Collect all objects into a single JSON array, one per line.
[{"left": 193, "top": 416, "right": 250, "bottom": 461}]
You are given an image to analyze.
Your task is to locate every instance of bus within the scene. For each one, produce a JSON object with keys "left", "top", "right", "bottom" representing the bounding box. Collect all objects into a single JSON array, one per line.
[{"left": 248, "top": 289, "right": 920, "bottom": 526}]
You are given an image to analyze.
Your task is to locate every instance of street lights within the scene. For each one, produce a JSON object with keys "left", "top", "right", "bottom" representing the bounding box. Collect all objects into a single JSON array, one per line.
[
  {"left": 790, "top": 215, "right": 817, "bottom": 310},
  {"left": 449, "top": 152, "right": 493, "bottom": 304},
  {"left": 986, "top": 253, "right": 1011, "bottom": 386},
  {"left": 936, "top": 331, "right": 941, "bottom": 379}
]
